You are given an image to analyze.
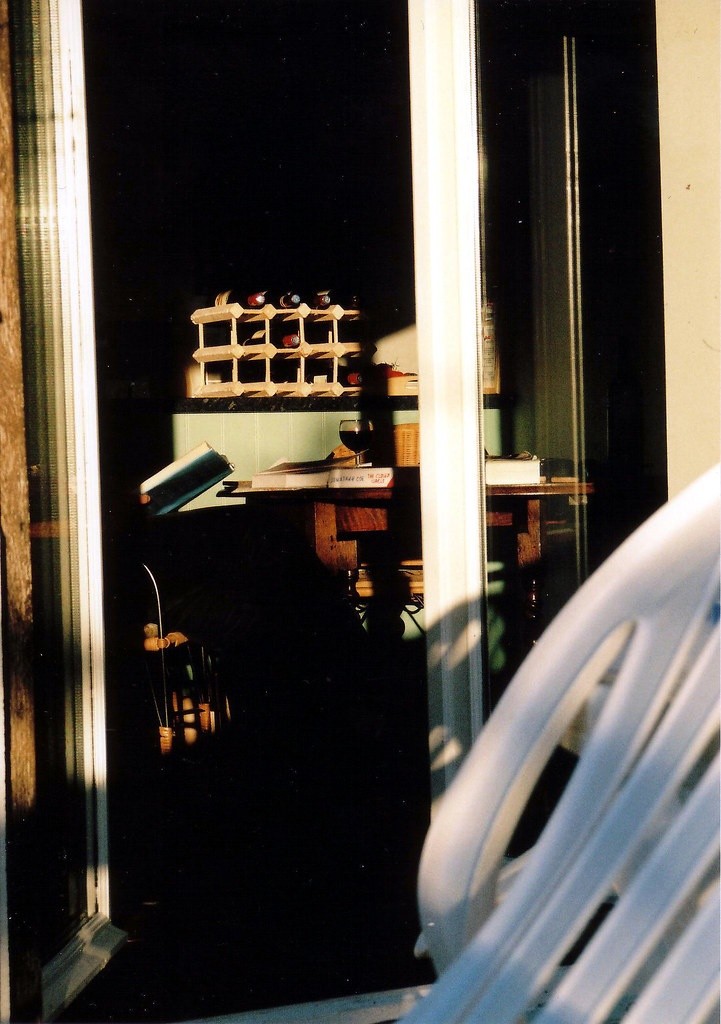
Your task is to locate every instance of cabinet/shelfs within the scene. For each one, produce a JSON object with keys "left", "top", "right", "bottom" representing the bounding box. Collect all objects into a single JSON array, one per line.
[{"left": 191, "top": 304, "right": 365, "bottom": 398}]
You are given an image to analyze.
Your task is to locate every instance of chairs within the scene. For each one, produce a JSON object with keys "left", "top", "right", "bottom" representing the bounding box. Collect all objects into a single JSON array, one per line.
[{"left": 323, "top": 422, "right": 426, "bottom": 636}]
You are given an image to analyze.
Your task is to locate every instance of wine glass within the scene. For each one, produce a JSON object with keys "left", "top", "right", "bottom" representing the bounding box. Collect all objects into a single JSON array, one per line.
[{"left": 338, "top": 419, "right": 376, "bottom": 469}]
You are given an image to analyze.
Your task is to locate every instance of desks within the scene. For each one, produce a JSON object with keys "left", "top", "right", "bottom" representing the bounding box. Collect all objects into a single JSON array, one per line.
[{"left": 217, "top": 478, "right": 592, "bottom": 672}]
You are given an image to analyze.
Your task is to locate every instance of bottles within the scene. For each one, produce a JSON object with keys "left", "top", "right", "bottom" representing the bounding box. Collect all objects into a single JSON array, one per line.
[
  {"left": 239, "top": 329, "right": 300, "bottom": 349},
  {"left": 271, "top": 293, "right": 301, "bottom": 308},
  {"left": 205, "top": 291, "right": 266, "bottom": 308},
  {"left": 307, "top": 373, "right": 362, "bottom": 387},
  {"left": 305, "top": 291, "right": 331, "bottom": 309}
]
